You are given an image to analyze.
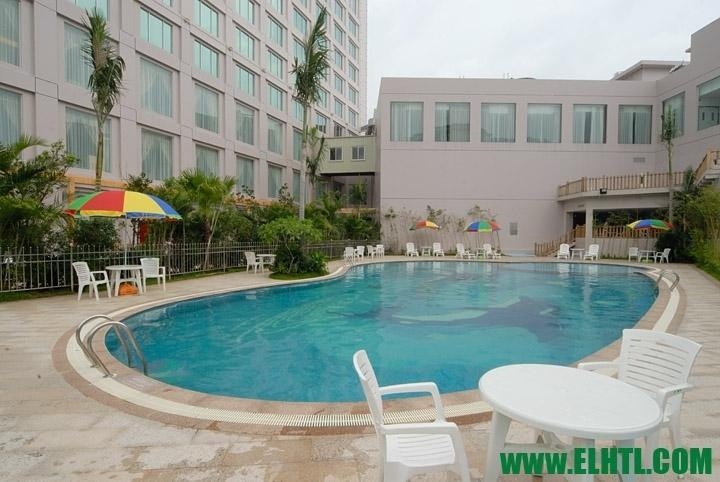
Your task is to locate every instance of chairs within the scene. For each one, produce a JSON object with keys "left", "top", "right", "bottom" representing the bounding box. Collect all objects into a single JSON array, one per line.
[
  {"left": 140, "top": 257, "right": 166, "bottom": 293},
  {"left": 344, "top": 242, "right": 500, "bottom": 266},
  {"left": 353, "top": 349, "right": 471, "bottom": 482},
  {"left": 577, "top": 329, "right": 703, "bottom": 482},
  {"left": 557, "top": 243, "right": 671, "bottom": 264},
  {"left": 72, "top": 261, "right": 112, "bottom": 302},
  {"left": 244, "top": 252, "right": 263, "bottom": 274}
]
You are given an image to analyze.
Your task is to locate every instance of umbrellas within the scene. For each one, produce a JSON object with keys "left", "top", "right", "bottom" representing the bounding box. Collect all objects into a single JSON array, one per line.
[
  {"left": 409, "top": 218, "right": 440, "bottom": 246},
  {"left": 463, "top": 220, "right": 503, "bottom": 248},
  {"left": 626, "top": 219, "right": 673, "bottom": 259},
  {"left": 62, "top": 186, "right": 183, "bottom": 284}
]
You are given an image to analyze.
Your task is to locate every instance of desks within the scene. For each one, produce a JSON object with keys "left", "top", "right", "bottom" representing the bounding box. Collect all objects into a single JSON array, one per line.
[
  {"left": 477, "top": 364, "right": 663, "bottom": 481},
  {"left": 256, "top": 254, "right": 277, "bottom": 272}
]
